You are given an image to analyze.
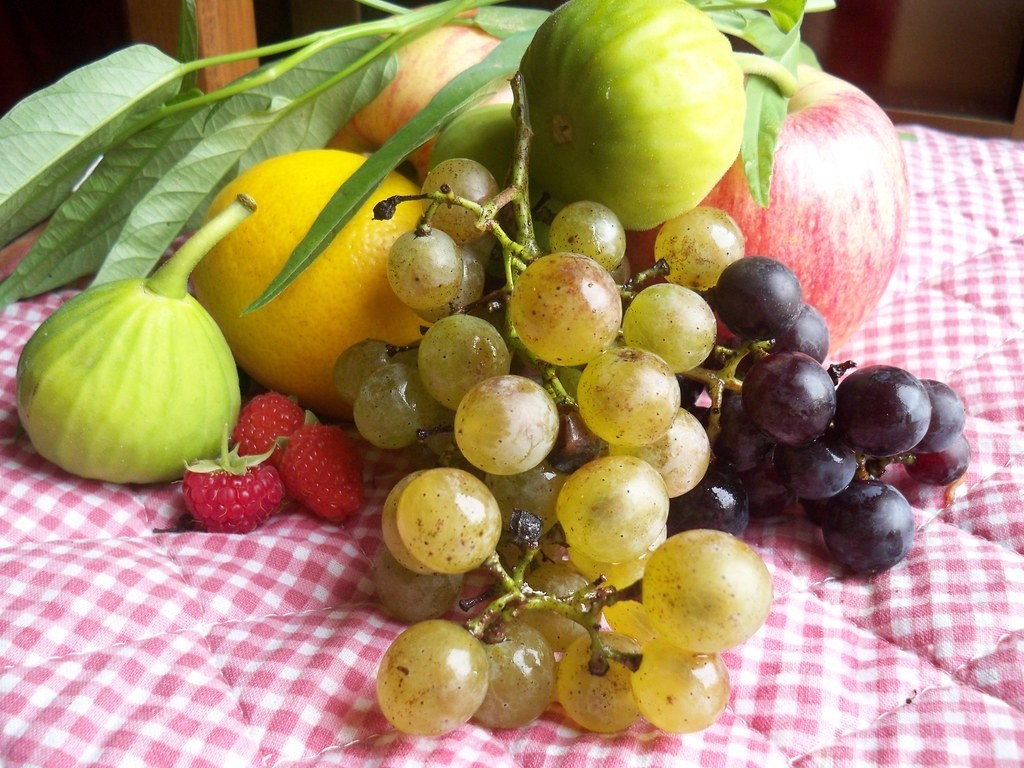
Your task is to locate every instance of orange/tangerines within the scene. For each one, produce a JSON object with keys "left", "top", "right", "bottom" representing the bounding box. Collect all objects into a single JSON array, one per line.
[{"left": 189, "top": 146, "right": 437, "bottom": 424}]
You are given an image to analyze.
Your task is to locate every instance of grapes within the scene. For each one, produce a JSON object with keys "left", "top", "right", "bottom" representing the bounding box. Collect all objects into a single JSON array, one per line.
[{"left": 332, "top": 69, "right": 970, "bottom": 741}]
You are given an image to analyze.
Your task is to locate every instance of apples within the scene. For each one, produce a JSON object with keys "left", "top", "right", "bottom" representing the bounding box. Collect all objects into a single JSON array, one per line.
[{"left": 322, "top": 7, "right": 519, "bottom": 192}]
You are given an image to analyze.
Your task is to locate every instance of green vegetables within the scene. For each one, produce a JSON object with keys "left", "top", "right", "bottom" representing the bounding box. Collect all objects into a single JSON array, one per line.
[{"left": 0, "top": 1, "right": 840, "bottom": 291}]
